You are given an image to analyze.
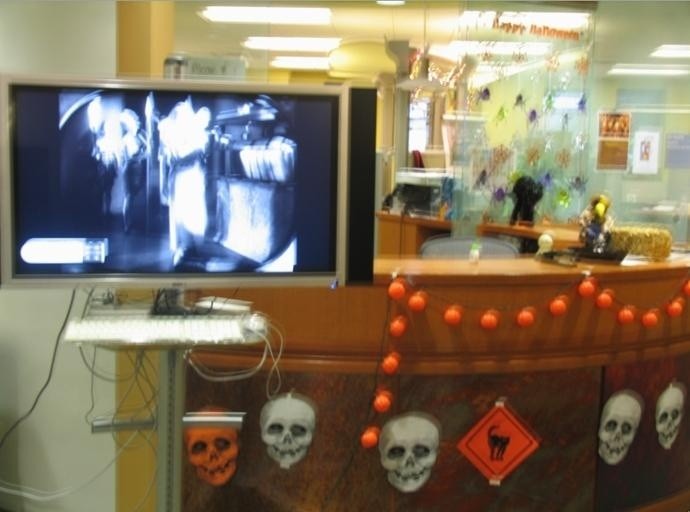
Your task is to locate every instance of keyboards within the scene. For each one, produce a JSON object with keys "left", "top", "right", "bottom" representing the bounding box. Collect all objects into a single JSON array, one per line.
[{"left": 65, "top": 314, "right": 247, "bottom": 344}]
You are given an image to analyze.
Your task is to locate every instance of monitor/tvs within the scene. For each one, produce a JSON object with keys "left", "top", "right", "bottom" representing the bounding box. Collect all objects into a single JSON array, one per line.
[{"left": 0, "top": 73, "right": 350, "bottom": 289}]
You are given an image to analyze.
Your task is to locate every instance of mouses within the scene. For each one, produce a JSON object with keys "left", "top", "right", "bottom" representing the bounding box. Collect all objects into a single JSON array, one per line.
[{"left": 244, "top": 312, "right": 265, "bottom": 331}]
